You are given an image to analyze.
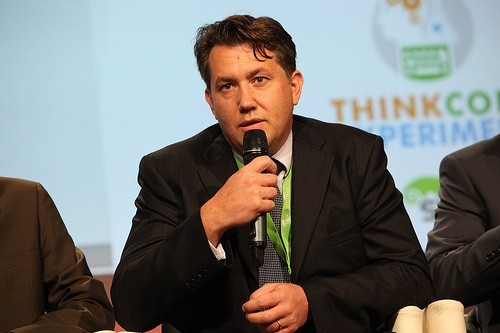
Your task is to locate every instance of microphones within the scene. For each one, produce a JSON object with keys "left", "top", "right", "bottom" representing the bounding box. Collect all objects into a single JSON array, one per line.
[{"left": 243, "top": 129, "right": 270, "bottom": 267}]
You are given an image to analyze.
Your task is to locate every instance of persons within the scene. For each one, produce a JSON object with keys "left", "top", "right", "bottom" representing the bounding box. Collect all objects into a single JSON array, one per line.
[
  {"left": 110, "top": 13, "right": 436, "bottom": 332},
  {"left": 0, "top": 176, "right": 116, "bottom": 332},
  {"left": 426, "top": 134, "right": 500, "bottom": 333}
]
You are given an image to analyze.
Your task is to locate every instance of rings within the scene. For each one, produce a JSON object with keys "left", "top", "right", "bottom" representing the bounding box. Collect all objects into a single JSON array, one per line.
[{"left": 276, "top": 319, "right": 285, "bottom": 328}]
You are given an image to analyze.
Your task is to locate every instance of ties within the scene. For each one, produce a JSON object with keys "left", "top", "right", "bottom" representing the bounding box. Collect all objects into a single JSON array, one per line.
[{"left": 260, "top": 170, "right": 293, "bottom": 312}]
